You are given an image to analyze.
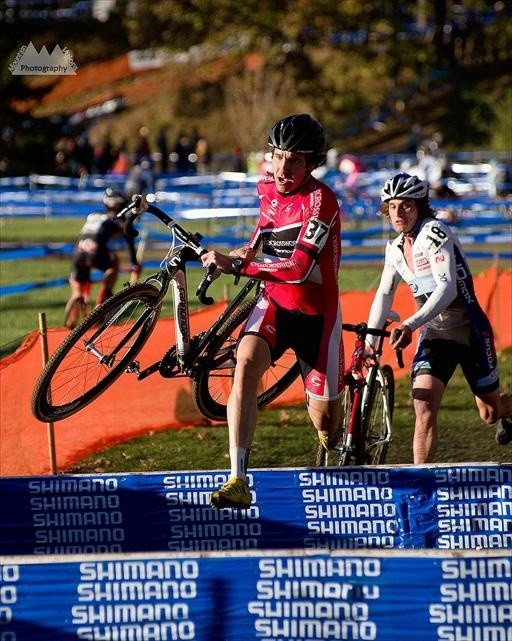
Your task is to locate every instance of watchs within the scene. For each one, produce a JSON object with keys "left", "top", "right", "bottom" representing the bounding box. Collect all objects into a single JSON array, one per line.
[{"left": 231, "top": 257, "right": 244, "bottom": 286}]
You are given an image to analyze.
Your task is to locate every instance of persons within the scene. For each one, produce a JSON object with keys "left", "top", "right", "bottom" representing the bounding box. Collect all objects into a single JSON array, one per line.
[
  {"left": 327, "top": 147, "right": 361, "bottom": 200},
  {"left": 1, "top": 103, "right": 275, "bottom": 225},
  {"left": 363, "top": 174, "right": 512, "bottom": 467},
  {"left": 200, "top": 113, "right": 347, "bottom": 509},
  {"left": 65, "top": 188, "right": 143, "bottom": 331},
  {"left": 401, "top": 120, "right": 476, "bottom": 223}
]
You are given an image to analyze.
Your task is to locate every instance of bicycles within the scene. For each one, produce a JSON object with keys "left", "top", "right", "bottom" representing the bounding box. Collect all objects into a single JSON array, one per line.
[{"left": 317, "top": 319, "right": 402, "bottom": 474}]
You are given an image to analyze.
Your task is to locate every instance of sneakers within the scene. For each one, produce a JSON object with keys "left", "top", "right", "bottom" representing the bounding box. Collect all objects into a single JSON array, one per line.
[
  {"left": 318, "top": 414, "right": 343, "bottom": 450},
  {"left": 211, "top": 477, "right": 252, "bottom": 509},
  {"left": 495, "top": 418, "right": 512, "bottom": 445}
]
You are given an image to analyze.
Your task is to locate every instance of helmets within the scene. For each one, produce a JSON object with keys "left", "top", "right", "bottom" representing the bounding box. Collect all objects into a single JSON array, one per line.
[
  {"left": 103, "top": 189, "right": 130, "bottom": 211},
  {"left": 268, "top": 114, "right": 326, "bottom": 154},
  {"left": 380, "top": 173, "right": 429, "bottom": 201}
]
